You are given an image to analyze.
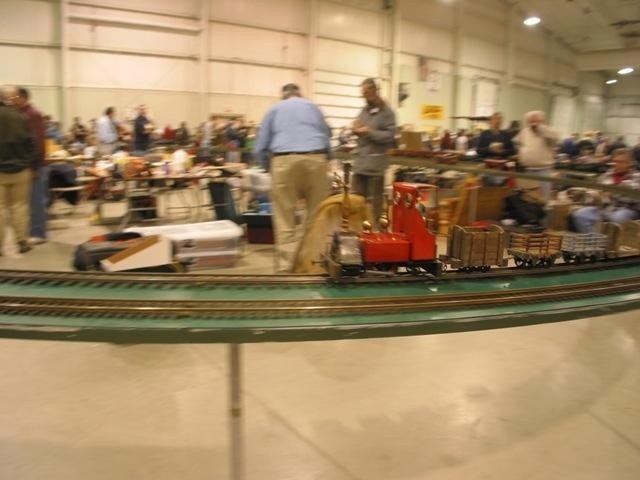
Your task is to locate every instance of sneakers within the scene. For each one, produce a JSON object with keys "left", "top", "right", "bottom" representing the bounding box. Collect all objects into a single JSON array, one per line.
[{"left": 16, "top": 235, "right": 47, "bottom": 252}]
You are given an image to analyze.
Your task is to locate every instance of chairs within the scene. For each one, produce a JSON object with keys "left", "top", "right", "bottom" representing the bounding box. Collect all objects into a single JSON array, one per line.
[{"left": 208, "top": 182, "right": 252, "bottom": 248}]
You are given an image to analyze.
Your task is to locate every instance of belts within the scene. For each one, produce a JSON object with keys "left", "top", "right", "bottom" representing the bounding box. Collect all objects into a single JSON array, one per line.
[{"left": 273, "top": 149, "right": 331, "bottom": 156}]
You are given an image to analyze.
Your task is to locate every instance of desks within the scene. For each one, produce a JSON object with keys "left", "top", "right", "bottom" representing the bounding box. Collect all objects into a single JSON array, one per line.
[{"left": 109, "top": 173, "right": 205, "bottom": 232}]
